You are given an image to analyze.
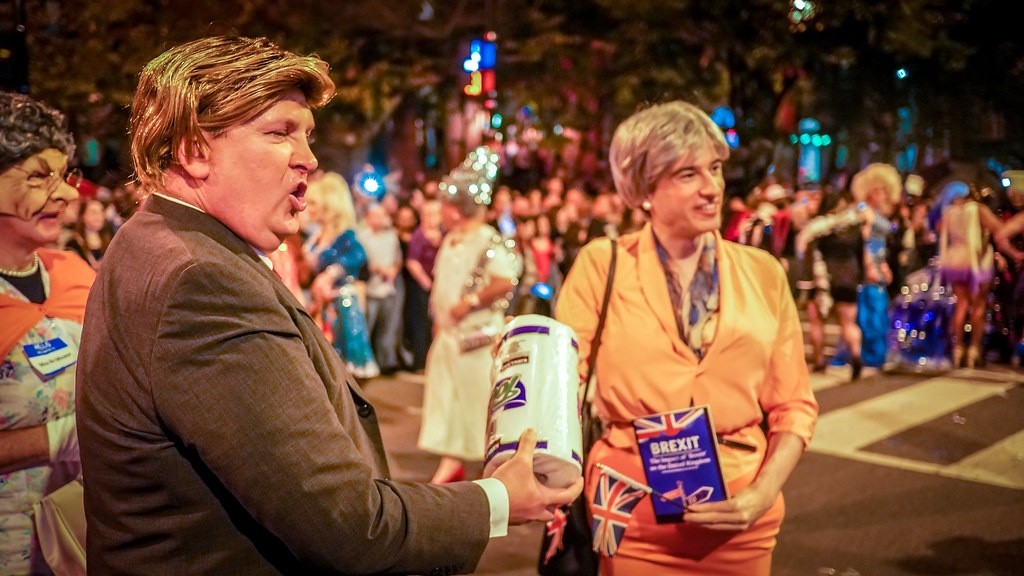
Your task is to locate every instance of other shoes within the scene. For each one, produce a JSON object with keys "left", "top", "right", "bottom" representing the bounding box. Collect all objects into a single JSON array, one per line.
[
  {"left": 852, "top": 356, "right": 864, "bottom": 383},
  {"left": 429, "top": 461, "right": 467, "bottom": 484}
]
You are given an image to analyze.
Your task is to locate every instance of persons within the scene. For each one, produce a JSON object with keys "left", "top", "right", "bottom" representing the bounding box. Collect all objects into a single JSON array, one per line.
[
  {"left": 555, "top": 101, "right": 819, "bottom": 576},
  {"left": 0, "top": 93, "right": 99, "bottom": 576},
  {"left": 0, "top": 127, "right": 1024, "bottom": 384},
  {"left": 74, "top": 37, "right": 584, "bottom": 576},
  {"left": 415, "top": 176, "right": 519, "bottom": 483}
]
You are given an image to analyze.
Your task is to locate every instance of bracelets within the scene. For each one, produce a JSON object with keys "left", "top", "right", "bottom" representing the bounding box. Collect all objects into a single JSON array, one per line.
[{"left": 465, "top": 289, "right": 482, "bottom": 309}]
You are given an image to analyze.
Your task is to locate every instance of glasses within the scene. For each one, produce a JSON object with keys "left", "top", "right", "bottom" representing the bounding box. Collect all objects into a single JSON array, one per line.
[{"left": 12, "top": 161, "right": 82, "bottom": 195}]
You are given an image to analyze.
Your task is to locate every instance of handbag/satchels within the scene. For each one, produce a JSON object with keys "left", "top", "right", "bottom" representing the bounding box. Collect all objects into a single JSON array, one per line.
[
  {"left": 458, "top": 322, "right": 496, "bottom": 351},
  {"left": 537, "top": 399, "right": 599, "bottom": 576}
]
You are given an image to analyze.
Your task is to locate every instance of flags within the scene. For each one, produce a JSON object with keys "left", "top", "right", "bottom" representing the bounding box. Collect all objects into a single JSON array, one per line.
[{"left": 591, "top": 467, "right": 652, "bottom": 558}]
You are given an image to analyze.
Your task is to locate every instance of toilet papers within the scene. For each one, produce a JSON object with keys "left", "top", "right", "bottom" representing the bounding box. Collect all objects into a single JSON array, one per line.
[{"left": 480, "top": 313, "right": 584, "bottom": 505}]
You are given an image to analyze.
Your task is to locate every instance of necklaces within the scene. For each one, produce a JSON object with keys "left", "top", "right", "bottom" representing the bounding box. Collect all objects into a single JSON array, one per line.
[{"left": 0, "top": 251, "right": 39, "bottom": 277}]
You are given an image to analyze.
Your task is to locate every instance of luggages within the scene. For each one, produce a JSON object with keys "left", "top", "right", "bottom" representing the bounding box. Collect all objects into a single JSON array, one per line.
[{"left": 894, "top": 285, "right": 956, "bottom": 374}]
